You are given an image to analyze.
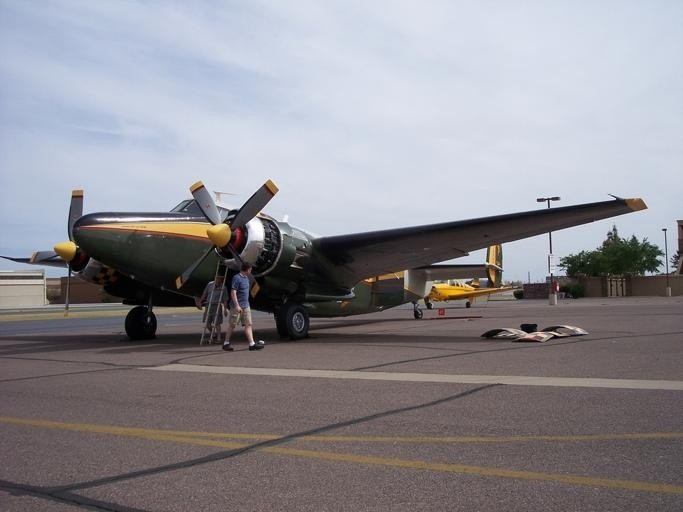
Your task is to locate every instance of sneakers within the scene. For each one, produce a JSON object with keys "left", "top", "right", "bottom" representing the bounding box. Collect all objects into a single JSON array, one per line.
[{"left": 208, "top": 337, "right": 264, "bottom": 351}]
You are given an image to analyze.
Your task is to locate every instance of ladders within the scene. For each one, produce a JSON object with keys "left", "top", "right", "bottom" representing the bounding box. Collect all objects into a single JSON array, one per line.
[{"left": 199, "top": 259, "right": 227, "bottom": 345}]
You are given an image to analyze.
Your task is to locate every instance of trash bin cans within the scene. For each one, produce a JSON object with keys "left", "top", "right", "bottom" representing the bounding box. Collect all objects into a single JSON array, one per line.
[
  {"left": 548, "top": 291, "right": 557, "bottom": 305},
  {"left": 665, "top": 287, "right": 671, "bottom": 296}
]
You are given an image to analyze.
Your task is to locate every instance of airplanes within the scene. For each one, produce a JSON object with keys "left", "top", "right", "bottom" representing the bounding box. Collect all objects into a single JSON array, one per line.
[
  {"left": 0, "top": 179, "right": 648, "bottom": 344},
  {"left": 424, "top": 278, "right": 519, "bottom": 310}
]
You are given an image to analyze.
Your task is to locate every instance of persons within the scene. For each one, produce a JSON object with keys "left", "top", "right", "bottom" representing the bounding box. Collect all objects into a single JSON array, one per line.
[{"left": 197, "top": 262, "right": 265, "bottom": 352}]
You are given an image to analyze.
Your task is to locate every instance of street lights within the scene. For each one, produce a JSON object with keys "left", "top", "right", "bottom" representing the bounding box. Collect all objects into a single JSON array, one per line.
[{"left": 662, "top": 228, "right": 671, "bottom": 297}]
